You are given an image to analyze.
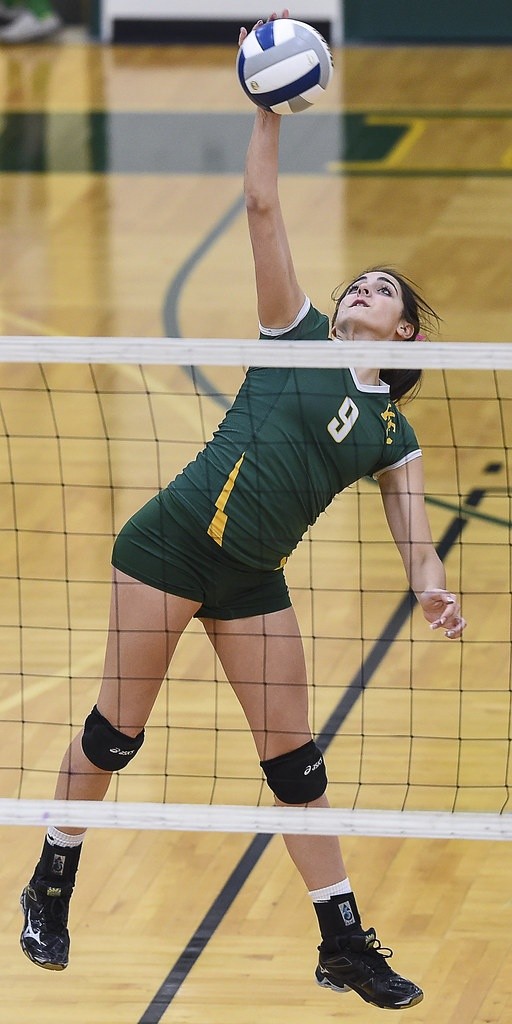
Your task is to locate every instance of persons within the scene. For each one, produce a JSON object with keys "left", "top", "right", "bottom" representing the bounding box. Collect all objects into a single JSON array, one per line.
[{"left": 18, "top": 8, "right": 473, "bottom": 1010}]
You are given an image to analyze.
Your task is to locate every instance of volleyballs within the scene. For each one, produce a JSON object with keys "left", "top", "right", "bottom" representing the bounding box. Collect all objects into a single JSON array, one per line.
[{"left": 235, "top": 18, "right": 336, "bottom": 116}]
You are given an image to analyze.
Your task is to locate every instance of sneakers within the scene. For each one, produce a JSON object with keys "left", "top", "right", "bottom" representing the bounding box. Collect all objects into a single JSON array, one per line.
[
  {"left": 314, "top": 928, "right": 423, "bottom": 1011},
  {"left": 18, "top": 872, "right": 71, "bottom": 971}
]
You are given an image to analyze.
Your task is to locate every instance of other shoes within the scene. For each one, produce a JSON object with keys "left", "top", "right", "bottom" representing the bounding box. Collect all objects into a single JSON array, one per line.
[{"left": 0, "top": 1, "right": 60, "bottom": 44}]
